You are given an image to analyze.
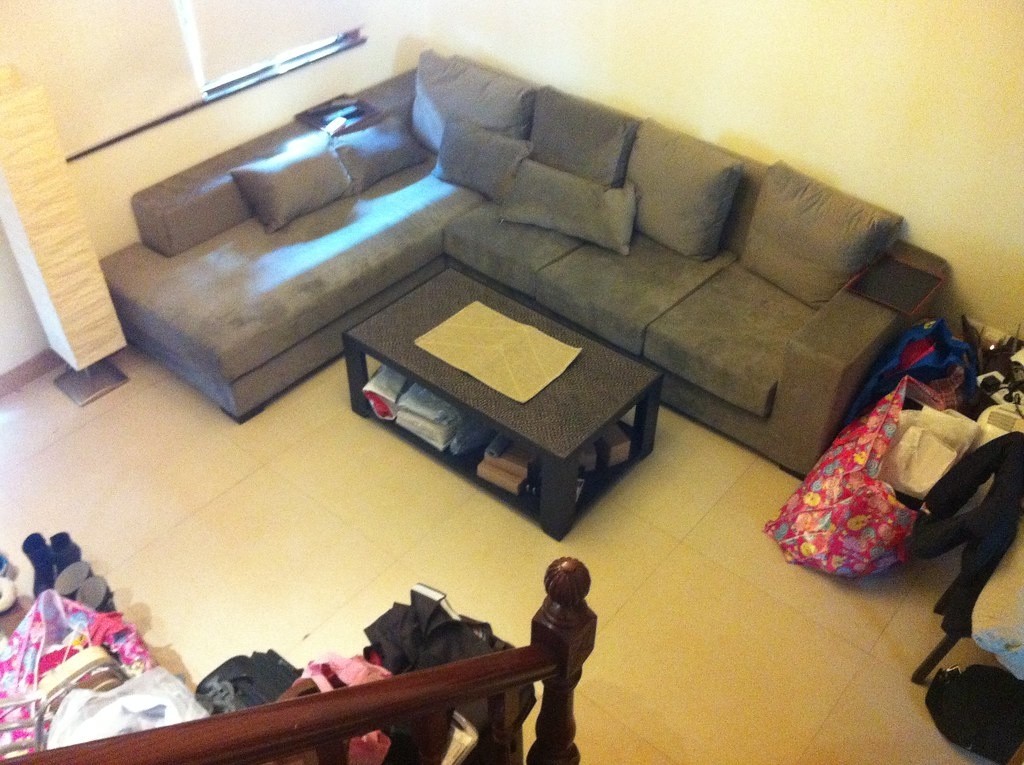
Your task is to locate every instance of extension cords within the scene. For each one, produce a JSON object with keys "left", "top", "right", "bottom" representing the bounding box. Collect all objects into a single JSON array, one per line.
[{"left": 976, "top": 370, "right": 1024, "bottom": 412}]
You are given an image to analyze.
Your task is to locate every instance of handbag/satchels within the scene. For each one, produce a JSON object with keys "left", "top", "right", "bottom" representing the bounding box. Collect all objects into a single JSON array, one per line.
[
  {"left": 762, "top": 375, "right": 980, "bottom": 580},
  {"left": 362, "top": 584, "right": 537, "bottom": 739},
  {"left": 0, "top": 589, "right": 396, "bottom": 765},
  {"left": 840, "top": 318, "right": 977, "bottom": 424},
  {"left": 362, "top": 363, "right": 414, "bottom": 421},
  {"left": 926, "top": 664, "right": 1024, "bottom": 765}
]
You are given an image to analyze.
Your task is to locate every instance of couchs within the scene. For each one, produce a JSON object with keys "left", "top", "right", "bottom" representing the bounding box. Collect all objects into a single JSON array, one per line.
[{"left": 97, "top": 54, "right": 950, "bottom": 482}]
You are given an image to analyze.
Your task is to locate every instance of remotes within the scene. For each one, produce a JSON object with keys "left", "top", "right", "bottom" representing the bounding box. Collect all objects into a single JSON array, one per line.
[{"left": 322, "top": 105, "right": 365, "bottom": 126}]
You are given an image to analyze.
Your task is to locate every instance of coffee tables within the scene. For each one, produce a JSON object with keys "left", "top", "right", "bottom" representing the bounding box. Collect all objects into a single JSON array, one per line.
[{"left": 339, "top": 264, "right": 665, "bottom": 544}]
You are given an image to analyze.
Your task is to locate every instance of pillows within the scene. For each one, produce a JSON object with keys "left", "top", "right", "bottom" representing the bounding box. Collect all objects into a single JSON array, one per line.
[{"left": 228, "top": 50, "right": 904, "bottom": 312}]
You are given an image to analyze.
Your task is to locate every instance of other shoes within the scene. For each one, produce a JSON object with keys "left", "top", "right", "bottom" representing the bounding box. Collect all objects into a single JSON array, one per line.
[
  {"left": 0, "top": 553, "right": 18, "bottom": 613},
  {"left": 22, "top": 533, "right": 54, "bottom": 600},
  {"left": 50, "top": 532, "right": 81, "bottom": 578}
]
